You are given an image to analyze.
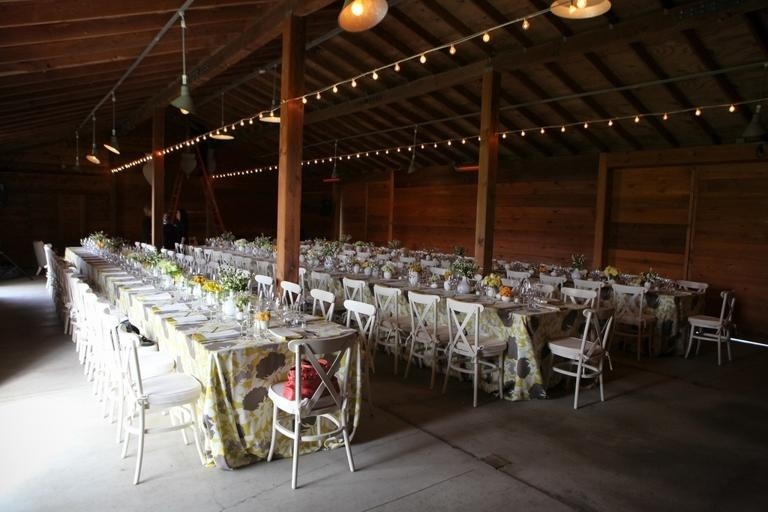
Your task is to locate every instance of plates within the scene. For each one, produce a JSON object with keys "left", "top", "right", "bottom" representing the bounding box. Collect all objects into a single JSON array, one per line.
[
  {"left": 454, "top": 295, "right": 479, "bottom": 300},
  {"left": 494, "top": 303, "right": 518, "bottom": 309}
]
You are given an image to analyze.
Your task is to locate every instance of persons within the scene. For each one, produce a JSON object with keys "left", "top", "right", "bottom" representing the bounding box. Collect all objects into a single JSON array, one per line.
[{"left": 141, "top": 207, "right": 189, "bottom": 250}]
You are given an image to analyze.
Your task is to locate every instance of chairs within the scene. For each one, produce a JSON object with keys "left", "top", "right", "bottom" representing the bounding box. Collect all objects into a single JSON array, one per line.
[
  {"left": 31, "top": 242, "right": 377, "bottom": 485},
  {"left": 542, "top": 271, "right": 708, "bottom": 410},
  {"left": 682, "top": 288, "right": 737, "bottom": 367},
  {"left": 374, "top": 246, "right": 568, "bottom": 408}
]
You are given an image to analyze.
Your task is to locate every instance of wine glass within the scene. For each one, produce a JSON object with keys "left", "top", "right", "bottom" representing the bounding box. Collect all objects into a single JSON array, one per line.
[
  {"left": 80, "top": 236, "right": 307, "bottom": 344},
  {"left": 519, "top": 278, "right": 546, "bottom": 313}
]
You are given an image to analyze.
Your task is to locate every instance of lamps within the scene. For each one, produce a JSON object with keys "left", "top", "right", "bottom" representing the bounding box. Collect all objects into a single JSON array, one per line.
[
  {"left": 550, "top": 0, "right": 611, "bottom": 21},
  {"left": 336, "top": 0, "right": 388, "bottom": 34},
  {"left": 72, "top": 10, "right": 280, "bottom": 166}
]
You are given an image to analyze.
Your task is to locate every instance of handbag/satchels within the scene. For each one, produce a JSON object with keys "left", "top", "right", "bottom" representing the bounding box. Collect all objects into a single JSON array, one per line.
[{"left": 283, "top": 358, "right": 340, "bottom": 400}]
